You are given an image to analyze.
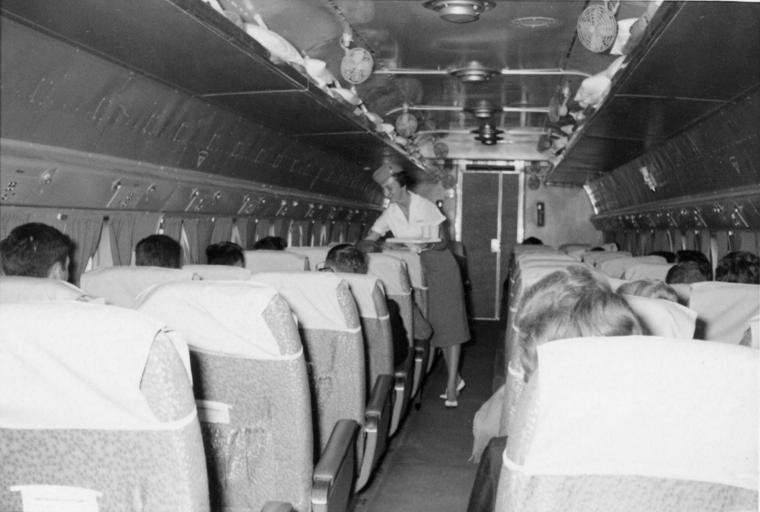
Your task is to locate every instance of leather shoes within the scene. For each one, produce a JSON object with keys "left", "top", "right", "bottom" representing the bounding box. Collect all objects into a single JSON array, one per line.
[
  {"left": 445, "top": 388, "right": 458, "bottom": 408},
  {"left": 440, "top": 380, "right": 465, "bottom": 399}
]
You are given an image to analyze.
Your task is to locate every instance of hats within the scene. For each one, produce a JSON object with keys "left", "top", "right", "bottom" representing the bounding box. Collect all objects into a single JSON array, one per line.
[{"left": 372, "top": 163, "right": 403, "bottom": 184}]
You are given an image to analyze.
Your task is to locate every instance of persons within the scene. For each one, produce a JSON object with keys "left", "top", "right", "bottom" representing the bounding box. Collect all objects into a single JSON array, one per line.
[
  {"left": 470, "top": 279, "right": 678, "bottom": 461},
  {"left": 322, "top": 244, "right": 368, "bottom": 273},
  {"left": 666, "top": 260, "right": 710, "bottom": 288},
  {"left": 205, "top": 240, "right": 246, "bottom": 266},
  {"left": 467, "top": 268, "right": 654, "bottom": 512},
  {"left": 363, "top": 162, "right": 471, "bottom": 407},
  {"left": 136, "top": 234, "right": 183, "bottom": 268},
  {"left": 0, "top": 223, "right": 71, "bottom": 282},
  {"left": 354, "top": 238, "right": 434, "bottom": 341},
  {"left": 716, "top": 249, "right": 760, "bottom": 286},
  {"left": 674, "top": 250, "right": 710, "bottom": 277}
]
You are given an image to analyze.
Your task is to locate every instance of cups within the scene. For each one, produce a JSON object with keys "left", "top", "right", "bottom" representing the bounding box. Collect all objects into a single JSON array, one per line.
[
  {"left": 431, "top": 226, "right": 439, "bottom": 239},
  {"left": 421, "top": 226, "right": 430, "bottom": 239}
]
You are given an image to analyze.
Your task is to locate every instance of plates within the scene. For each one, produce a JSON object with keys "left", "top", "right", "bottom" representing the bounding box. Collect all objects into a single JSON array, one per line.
[{"left": 385, "top": 238, "right": 442, "bottom": 243}]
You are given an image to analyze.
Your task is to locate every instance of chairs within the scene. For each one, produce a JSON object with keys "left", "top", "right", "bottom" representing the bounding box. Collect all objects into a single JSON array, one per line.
[
  {"left": 178, "top": 259, "right": 255, "bottom": 283},
  {"left": 79, "top": 266, "right": 193, "bottom": 308},
  {"left": 355, "top": 253, "right": 431, "bottom": 410},
  {"left": 515, "top": 248, "right": 563, "bottom": 259},
  {"left": 576, "top": 251, "right": 633, "bottom": 268},
  {"left": 520, "top": 259, "right": 588, "bottom": 277},
  {"left": 136, "top": 280, "right": 357, "bottom": 511},
  {"left": 514, "top": 243, "right": 556, "bottom": 254},
  {"left": 236, "top": 248, "right": 307, "bottom": 281},
  {"left": 490, "top": 334, "right": 754, "bottom": 512},
  {"left": 2, "top": 293, "right": 210, "bottom": 512},
  {"left": 679, "top": 280, "right": 755, "bottom": 342},
  {"left": 286, "top": 244, "right": 339, "bottom": 274},
  {"left": 566, "top": 242, "right": 616, "bottom": 259},
  {"left": 520, "top": 253, "right": 569, "bottom": 268},
  {"left": 621, "top": 264, "right": 681, "bottom": 280},
  {"left": 597, "top": 255, "right": 673, "bottom": 280},
  {"left": 2, "top": 272, "right": 88, "bottom": 310},
  {"left": 519, "top": 266, "right": 586, "bottom": 292},
  {"left": 253, "top": 272, "right": 382, "bottom": 491},
  {"left": 308, "top": 267, "right": 412, "bottom": 437},
  {"left": 364, "top": 247, "right": 433, "bottom": 346}
]
